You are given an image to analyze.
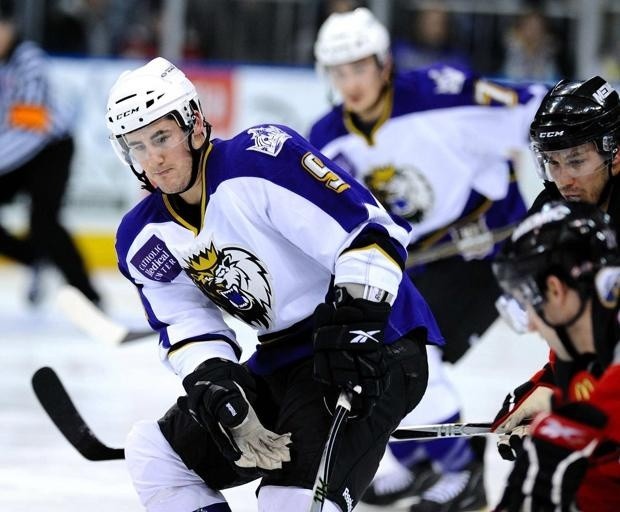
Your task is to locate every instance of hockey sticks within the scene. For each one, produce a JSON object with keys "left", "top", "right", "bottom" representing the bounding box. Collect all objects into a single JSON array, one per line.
[
  {"left": 57, "top": 208, "right": 519, "bottom": 352},
  {"left": 31, "top": 366, "right": 520, "bottom": 462}
]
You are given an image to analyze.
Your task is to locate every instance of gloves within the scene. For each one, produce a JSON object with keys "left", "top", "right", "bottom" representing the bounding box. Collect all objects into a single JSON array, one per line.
[
  {"left": 320, "top": 298, "right": 388, "bottom": 420},
  {"left": 182, "top": 357, "right": 295, "bottom": 469}
]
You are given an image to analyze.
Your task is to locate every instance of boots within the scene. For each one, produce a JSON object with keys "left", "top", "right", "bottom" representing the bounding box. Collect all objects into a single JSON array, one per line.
[{"left": 361, "top": 435, "right": 487, "bottom": 510}]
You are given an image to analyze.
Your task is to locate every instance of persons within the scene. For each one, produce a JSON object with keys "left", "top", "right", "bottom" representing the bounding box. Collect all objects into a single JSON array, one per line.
[
  {"left": 493, "top": 73, "right": 619, "bottom": 462},
  {"left": 300, "top": 4, "right": 559, "bottom": 510},
  {"left": 103, "top": 54, "right": 446, "bottom": 510},
  {"left": 31, "top": 0, "right": 619, "bottom": 92},
  {"left": 491, "top": 194, "right": 619, "bottom": 511},
  {"left": 0, "top": 1, "right": 112, "bottom": 317}
]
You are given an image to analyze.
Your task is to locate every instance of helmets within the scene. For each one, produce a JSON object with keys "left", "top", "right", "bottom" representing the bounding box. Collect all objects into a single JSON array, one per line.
[
  {"left": 527, "top": 76, "right": 618, "bottom": 181},
  {"left": 106, "top": 57, "right": 203, "bottom": 166},
  {"left": 489, "top": 200, "right": 608, "bottom": 297},
  {"left": 313, "top": 8, "right": 391, "bottom": 75}
]
